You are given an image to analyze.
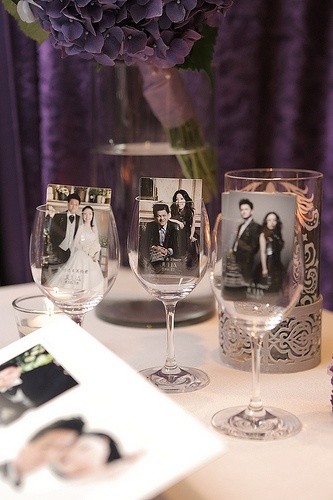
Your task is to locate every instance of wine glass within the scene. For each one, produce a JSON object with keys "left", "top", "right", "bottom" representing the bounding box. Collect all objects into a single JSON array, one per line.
[
  {"left": 210, "top": 214, "right": 305, "bottom": 440},
  {"left": 127, "top": 196, "right": 211, "bottom": 393},
  {"left": 29, "top": 204, "right": 120, "bottom": 326}
]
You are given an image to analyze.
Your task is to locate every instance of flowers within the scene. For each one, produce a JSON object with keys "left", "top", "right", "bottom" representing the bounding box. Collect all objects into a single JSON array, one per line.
[{"left": 1, "top": 0, "right": 233, "bottom": 205}]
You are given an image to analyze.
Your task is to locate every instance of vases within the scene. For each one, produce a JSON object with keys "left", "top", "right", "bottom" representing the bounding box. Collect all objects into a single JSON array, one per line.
[{"left": 92, "top": 66, "right": 218, "bottom": 330}]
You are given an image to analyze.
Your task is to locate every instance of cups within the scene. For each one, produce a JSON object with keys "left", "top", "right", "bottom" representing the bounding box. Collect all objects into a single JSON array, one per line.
[
  {"left": 220, "top": 169, "right": 323, "bottom": 372},
  {"left": 12, "top": 295, "right": 81, "bottom": 339}
]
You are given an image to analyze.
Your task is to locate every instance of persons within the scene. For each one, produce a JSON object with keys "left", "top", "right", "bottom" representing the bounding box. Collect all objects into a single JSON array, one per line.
[
  {"left": 0, "top": 360, "right": 78, "bottom": 429},
  {"left": 141, "top": 204, "right": 184, "bottom": 276},
  {"left": 70, "top": 206, "right": 104, "bottom": 290},
  {"left": 0, "top": 418, "right": 85, "bottom": 499},
  {"left": 19, "top": 431, "right": 122, "bottom": 495},
  {"left": 47, "top": 193, "right": 83, "bottom": 263},
  {"left": 229, "top": 200, "right": 263, "bottom": 283},
  {"left": 259, "top": 212, "right": 286, "bottom": 295},
  {"left": 169, "top": 189, "right": 198, "bottom": 269}
]
